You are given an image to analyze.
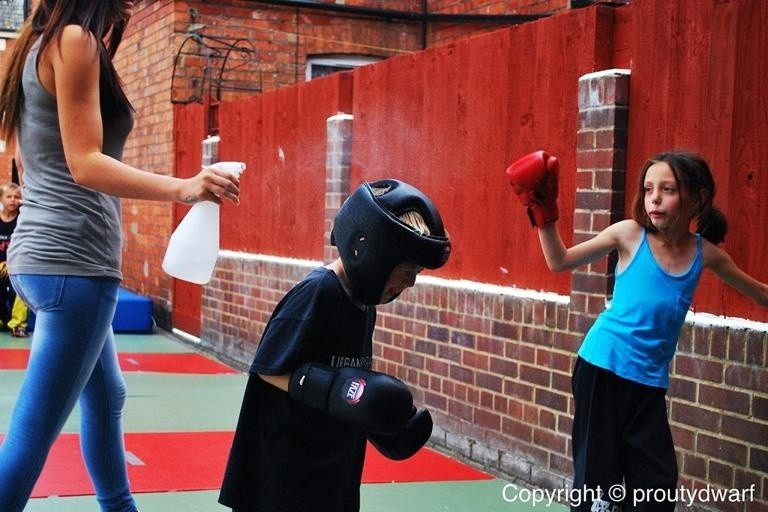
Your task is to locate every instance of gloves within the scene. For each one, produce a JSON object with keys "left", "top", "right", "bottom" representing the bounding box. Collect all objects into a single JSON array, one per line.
[
  {"left": 507, "top": 151, "right": 559, "bottom": 228},
  {"left": 365, "top": 408, "right": 433, "bottom": 461},
  {"left": 289, "top": 360, "right": 413, "bottom": 436}
]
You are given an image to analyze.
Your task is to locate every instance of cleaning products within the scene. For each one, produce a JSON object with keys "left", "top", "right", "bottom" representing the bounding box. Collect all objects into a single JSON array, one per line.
[{"left": 161, "top": 161, "right": 247, "bottom": 286}]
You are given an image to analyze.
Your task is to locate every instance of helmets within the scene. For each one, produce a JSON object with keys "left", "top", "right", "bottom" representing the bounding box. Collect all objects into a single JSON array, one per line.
[{"left": 330, "top": 180, "right": 451, "bottom": 305}]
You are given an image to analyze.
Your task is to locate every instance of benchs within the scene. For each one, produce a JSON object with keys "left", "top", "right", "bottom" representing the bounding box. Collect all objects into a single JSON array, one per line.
[{"left": 109, "top": 284, "right": 151, "bottom": 334}]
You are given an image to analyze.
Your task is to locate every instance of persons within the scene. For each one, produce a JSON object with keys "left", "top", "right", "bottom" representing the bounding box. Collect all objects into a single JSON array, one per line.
[
  {"left": 216, "top": 178, "right": 452, "bottom": 512},
  {"left": 8, "top": 294, "right": 29, "bottom": 337},
  {"left": 0, "top": 181, "right": 31, "bottom": 330},
  {"left": 0, "top": 0, "right": 242, "bottom": 512},
  {"left": 504, "top": 150, "right": 768, "bottom": 510}
]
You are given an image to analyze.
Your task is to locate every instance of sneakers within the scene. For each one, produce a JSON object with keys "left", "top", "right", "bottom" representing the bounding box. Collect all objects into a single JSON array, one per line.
[{"left": 10, "top": 326, "right": 28, "bottom": 337}]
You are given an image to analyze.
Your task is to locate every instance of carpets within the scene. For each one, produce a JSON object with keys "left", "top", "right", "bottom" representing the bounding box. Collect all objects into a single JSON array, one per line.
[
  {"left": 0, "top": 431, "right": 496, "bottom": 499},
  {"left": 0, "top": 343, "right": 237, "bottom": 378}
]
